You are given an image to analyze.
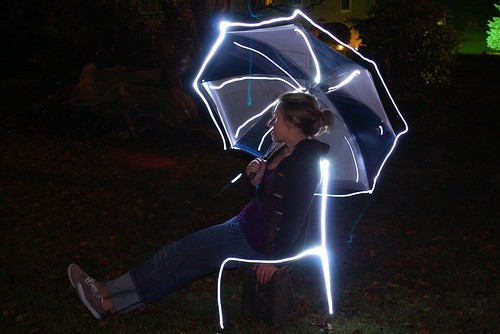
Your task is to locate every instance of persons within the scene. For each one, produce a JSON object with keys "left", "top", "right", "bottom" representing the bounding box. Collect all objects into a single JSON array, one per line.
[{"left": 68, "top": 93, "right": 336, "bottom": 320}]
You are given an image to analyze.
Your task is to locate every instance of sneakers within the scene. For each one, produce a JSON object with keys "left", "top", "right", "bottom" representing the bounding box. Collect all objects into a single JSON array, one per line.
[
  {"left": 76, "top": 283, "right": 105, "bottom": 317},
  {"left": 67, "top": 263, "right": 97, "bottom": 299}
]
